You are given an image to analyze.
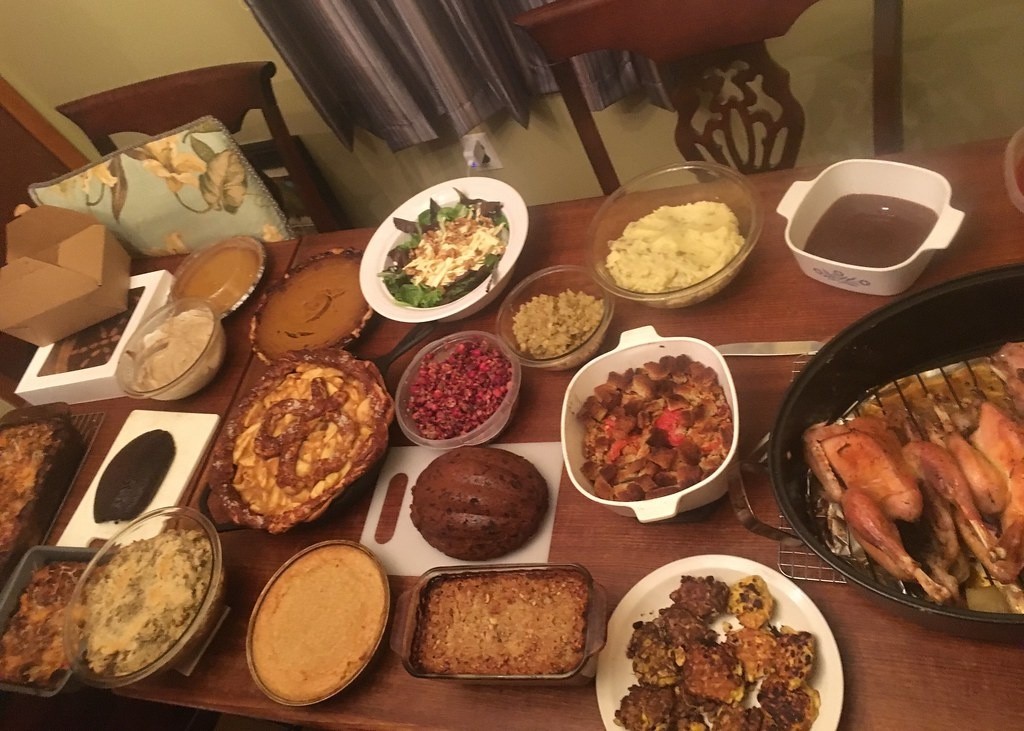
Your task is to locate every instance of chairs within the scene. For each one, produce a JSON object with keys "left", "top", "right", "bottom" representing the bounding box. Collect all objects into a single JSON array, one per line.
[
  {"left": 56, "top": 61, "right": 352, "bottom": 234},
  {"left": 514, "top": 1, "right": 905, "bottom": 195}
]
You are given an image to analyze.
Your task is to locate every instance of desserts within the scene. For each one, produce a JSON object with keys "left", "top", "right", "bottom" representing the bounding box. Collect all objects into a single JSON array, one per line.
[
  {"left": 607, "top": 201, "right": 744, "bottom": 300},
  {"left": 252, "top": 542, "right": 387, "bottom": 702}
]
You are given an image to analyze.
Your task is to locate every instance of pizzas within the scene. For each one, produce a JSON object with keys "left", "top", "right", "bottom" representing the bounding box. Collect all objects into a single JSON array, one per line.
[{"left": 208, "top": 352, "right": 392, "bottom": 533}]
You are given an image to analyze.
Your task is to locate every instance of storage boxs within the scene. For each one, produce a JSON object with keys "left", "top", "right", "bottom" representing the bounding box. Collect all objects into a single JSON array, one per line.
[
  {"left": 13, "top": 269, "right": 174, "bottom": 409},
  {"left": 0, "top": 205, "right": 132, "bottom": 348}
]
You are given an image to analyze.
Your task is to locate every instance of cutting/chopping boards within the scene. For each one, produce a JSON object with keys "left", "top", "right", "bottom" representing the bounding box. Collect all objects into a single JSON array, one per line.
[
  {"left": 359, "top": 441, "right": 566, "bottom": 576},
  {"left": 54, "top": 410, "right": 220, "bottom": 555},
  {"left": 249, "top": 246, "right": 374, "bottom": 368}
]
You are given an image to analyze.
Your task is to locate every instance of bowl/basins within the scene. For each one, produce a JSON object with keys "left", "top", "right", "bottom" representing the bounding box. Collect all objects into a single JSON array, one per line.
[
  {"left": 0, "top": 545, "right": 101, "bottom": 708},
  {"left": 496, "top": 264, "right": 614, "bottom": 372},
  {"left": 774, "top": 158, "right": 965, "bottom": 296},
  {"left": 116, "top": 297, "right": 226, "bottom": 401},
  {"left": 560, "top": 325, "right": 741, "bottom": 523},
  {"left": 0, "top": 402, "right": 87, "bottom": 584},
  {"left": 584, "top": 160, "right": 762, "bottom": 308},
  {"left": 245, "top": 538, "right": 391, "bottom": 706},
  {"left": 389, "top": 562, "right": 608, "bottom": 686},
  {"left": 1003, "top": 125, "right": 1024, "bottom": 214},
  {"left": 358, "top": 177, "right": 529, "bottom": 323},
  {"left": 62, "top": 505, "right": 222, "bottom": 689},
  {"left": 169, "top": 236, "right": 266, "bottom": 320},
  {"left": 395, "top": 330, "right": 521, "bottom": 451}
]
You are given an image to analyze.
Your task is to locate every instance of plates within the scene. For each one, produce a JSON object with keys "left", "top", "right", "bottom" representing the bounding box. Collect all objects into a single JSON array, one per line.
[{"left": 594, "top": 554, "right": 844, "bottom": 731}]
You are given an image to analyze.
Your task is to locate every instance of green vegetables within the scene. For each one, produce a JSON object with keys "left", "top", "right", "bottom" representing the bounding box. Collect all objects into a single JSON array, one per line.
[
  {"left": 419, "top": 204, "right": 508, "bottom": 228},
  {"left": 381, "top": 272, "right": 442, "bottom": 308}
]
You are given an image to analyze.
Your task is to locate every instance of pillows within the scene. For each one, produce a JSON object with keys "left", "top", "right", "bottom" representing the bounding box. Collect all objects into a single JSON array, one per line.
[{"left": 25, "top": 115, "right": 295, "bottom": 257}]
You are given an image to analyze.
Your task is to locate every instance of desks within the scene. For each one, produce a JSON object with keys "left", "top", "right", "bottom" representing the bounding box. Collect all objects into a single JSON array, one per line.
[{"left": 0, "top": 137, "right": 1024, "bottom": 731}]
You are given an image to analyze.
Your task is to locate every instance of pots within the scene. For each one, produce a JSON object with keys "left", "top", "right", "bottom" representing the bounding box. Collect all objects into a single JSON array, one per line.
[
  {"left": 199, "top": 321, "right": 435, "bottom": 537},
  {"left": 729, "top": 263, "right": 1024, "bottom": 624}
]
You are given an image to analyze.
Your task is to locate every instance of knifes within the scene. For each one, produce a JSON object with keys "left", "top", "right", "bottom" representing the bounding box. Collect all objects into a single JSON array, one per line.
[{"left": 714, "top": 341, "right": 827, "bottom": 355}]
